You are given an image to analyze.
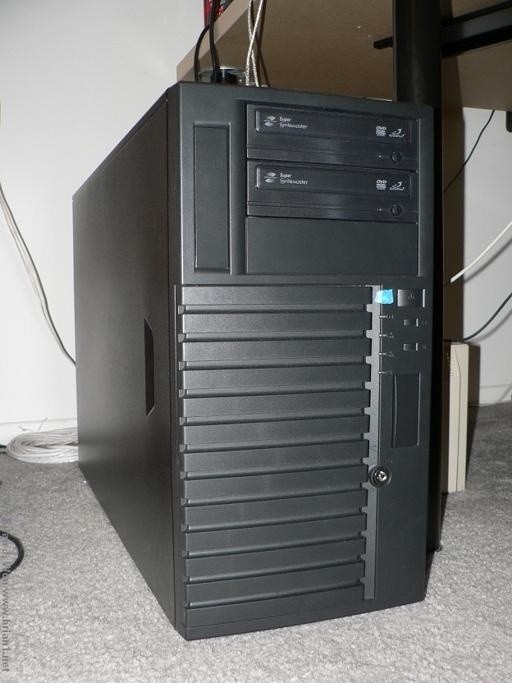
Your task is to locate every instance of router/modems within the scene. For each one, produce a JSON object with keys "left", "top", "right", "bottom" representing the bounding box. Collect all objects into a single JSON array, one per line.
[{"left": 440, "top": 340, "right": 469, "bottom": 495}]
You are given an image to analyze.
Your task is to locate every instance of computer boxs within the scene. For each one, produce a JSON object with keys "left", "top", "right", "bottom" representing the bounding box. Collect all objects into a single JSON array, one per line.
[{"left": 73, "top": 79, "right": 434, "bottom": 643}]
